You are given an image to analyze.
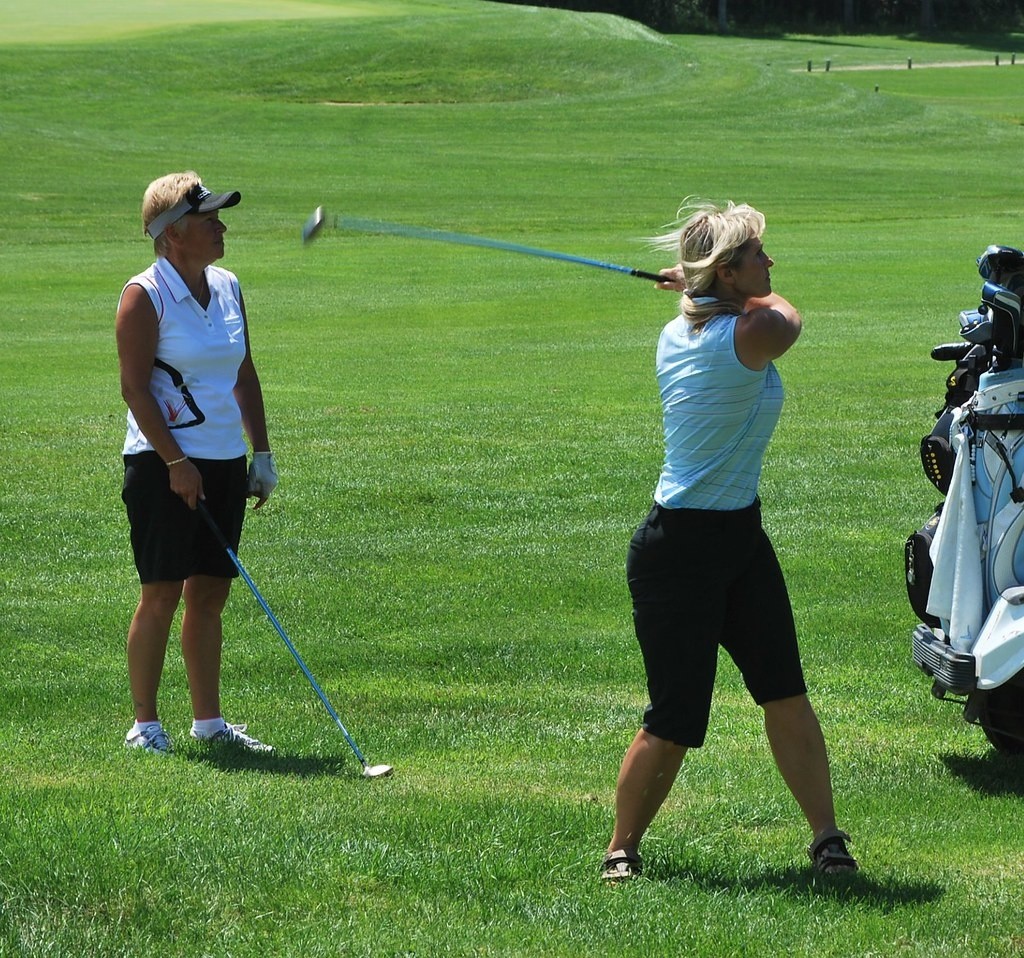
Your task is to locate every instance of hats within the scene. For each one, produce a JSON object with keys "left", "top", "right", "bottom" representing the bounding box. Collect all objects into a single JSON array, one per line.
[{"left": 147, "top": 184, "right": 241, "bottom": 238}]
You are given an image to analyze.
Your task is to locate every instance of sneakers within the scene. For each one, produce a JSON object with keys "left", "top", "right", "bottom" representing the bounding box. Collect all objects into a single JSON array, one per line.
[
  {"left": 601, "top": 850, "right": 642, "bottom": 880},
  {"left": 808, "top": 830, "right": 858, "bottom": 875},
  {"left": 125, "top": 729, "right": 174, "bottom": 757},
  {"left": 190, "top": 722, "right": 275, "bottom": 753}
]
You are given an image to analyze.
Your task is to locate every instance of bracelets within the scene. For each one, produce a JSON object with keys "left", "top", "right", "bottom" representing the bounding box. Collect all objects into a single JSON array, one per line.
[{"left": 166, "top": 455, "right": 187, "bottom": 466}]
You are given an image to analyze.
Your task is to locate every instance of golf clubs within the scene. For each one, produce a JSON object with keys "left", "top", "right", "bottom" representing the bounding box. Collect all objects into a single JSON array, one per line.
[
  {"left": 196, "top": 497, "right": 395, "bottom": 780},
  {"left": 300, "top": 204, "right": 675, "bottom": 282},
  {"left": 930, "top": 239, "right": 1024, "bottom": 408}
]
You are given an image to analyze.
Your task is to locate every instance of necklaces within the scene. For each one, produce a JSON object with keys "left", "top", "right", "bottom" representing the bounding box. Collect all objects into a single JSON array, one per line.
[{"left": 195, "top": 283, "right": 204, "bottom": 303}]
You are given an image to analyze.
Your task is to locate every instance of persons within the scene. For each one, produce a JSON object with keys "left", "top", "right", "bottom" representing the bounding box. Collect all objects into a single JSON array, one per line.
[
  {"left": 599, "top": 197, "right": 859, "bottom": 884},
  {"left": 115, "top": 173, "right": 279, "bottom": 754}
]
